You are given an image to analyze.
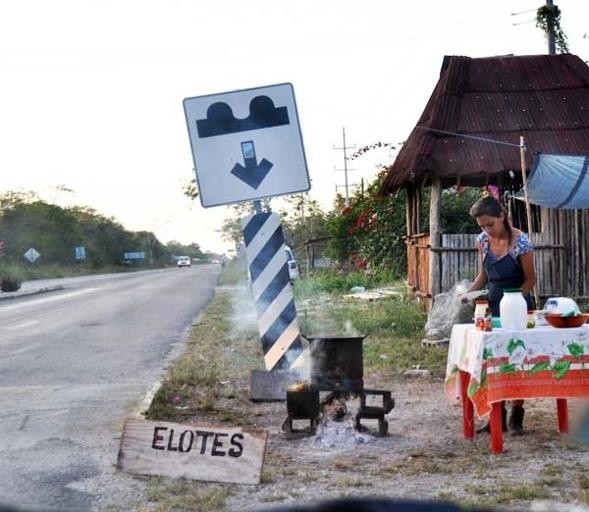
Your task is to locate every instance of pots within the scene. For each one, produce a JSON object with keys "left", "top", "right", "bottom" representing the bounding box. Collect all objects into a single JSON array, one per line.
[{"left": 300, "top": 333, "right": 370, "bottom": 393}]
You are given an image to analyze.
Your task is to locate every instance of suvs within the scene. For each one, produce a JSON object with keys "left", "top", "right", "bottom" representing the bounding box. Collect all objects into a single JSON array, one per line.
[{"left": 178, "top": 255, "right": 191, "bottom": 267}]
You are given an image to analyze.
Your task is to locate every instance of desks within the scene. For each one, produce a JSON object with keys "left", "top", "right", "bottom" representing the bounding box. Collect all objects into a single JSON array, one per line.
[{"left": 445, "top": 318, "right": 589, "bottom": 453}]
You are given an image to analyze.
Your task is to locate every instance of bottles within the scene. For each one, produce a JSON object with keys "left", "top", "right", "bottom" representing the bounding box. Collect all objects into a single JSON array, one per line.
[{"left": 474, "top": 301, "right": 493, "bottom": 332}]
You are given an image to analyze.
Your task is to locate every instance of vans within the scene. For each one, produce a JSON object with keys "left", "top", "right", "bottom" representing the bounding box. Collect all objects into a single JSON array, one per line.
[{"left": 244, "top": 239, "right": 302, "bottom": 289}]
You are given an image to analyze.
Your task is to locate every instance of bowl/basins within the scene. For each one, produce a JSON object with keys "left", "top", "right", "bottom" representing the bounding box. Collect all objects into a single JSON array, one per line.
[{"left": 544, "top": 313, "right": 589, "bottom": 328}]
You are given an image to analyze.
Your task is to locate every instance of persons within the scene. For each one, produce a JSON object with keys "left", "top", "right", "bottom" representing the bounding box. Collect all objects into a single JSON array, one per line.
[{"left": 456, "top": 196, "right": 539, "bottom": 437}]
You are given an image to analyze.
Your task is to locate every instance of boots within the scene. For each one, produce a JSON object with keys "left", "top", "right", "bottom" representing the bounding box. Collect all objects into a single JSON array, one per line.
[
  {"left": 475, "top": 408, "right": 507, "bottom": 433},
  {"left": 509, "top": 406, "right": 524, "bottom": 437}
]
